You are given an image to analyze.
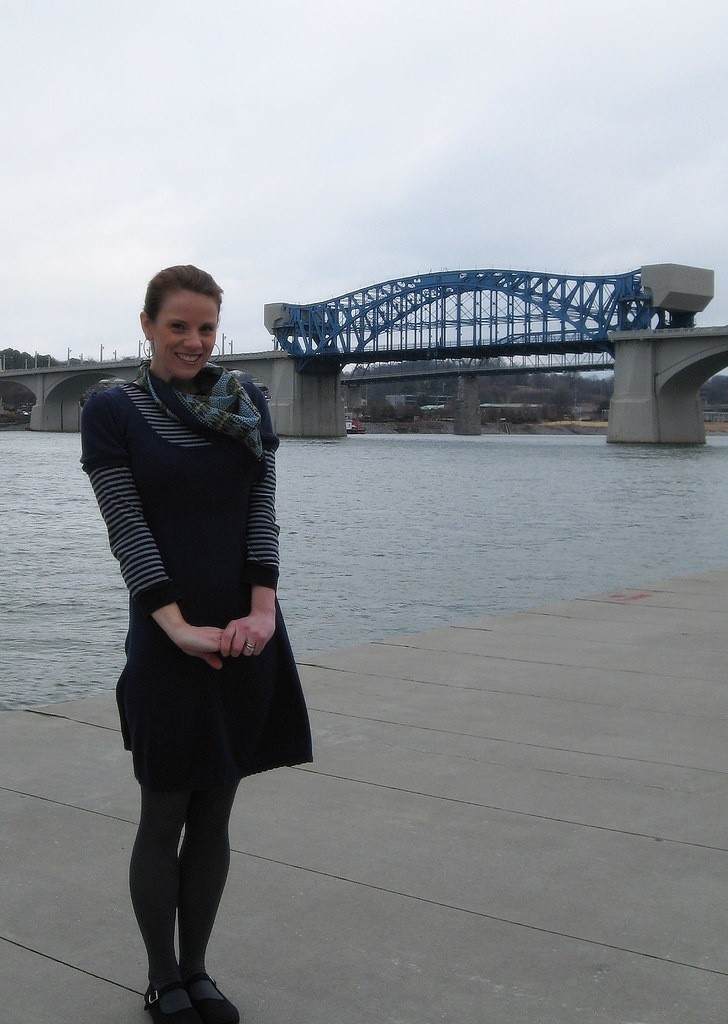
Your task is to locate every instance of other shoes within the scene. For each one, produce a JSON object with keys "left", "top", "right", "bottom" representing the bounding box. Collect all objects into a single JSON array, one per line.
[
  {"left": 143, "top": 979, "right": 196, "bottom": 1024},
  {"left": 184, "top": 971, "right": 240, "bottom": 1024}
]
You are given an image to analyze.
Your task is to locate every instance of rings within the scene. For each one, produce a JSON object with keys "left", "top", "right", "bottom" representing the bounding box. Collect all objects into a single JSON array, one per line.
[{"left": 245, "top": 643, "right": 255, "bottom": 651}]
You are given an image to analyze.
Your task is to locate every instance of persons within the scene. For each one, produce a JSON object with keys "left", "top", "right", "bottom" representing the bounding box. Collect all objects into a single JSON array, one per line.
[{"left": 81, "top": 264, "right": 313, "bottom": 1024}]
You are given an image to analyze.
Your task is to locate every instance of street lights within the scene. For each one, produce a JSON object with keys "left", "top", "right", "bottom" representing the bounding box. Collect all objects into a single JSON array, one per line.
[
  {"left": 100, "top": 344, "right": 104, "bottom": 361},
  {"left": 138, "top": 340, "right": 143, "bottom": 357},
  {"left": 67, "top": 347, "right": 72, "bottom": 366},
  {"left": 221, "top": 333, "right": 226, "bottom": 356},
  {"left": 112, "top": 350, "right": 117, "bottom": 366}
]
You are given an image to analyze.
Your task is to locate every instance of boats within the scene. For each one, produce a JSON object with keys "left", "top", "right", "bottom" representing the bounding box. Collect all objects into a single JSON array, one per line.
[{"left": 344, "top": 416, "right": 366, "bottom": 434}]
